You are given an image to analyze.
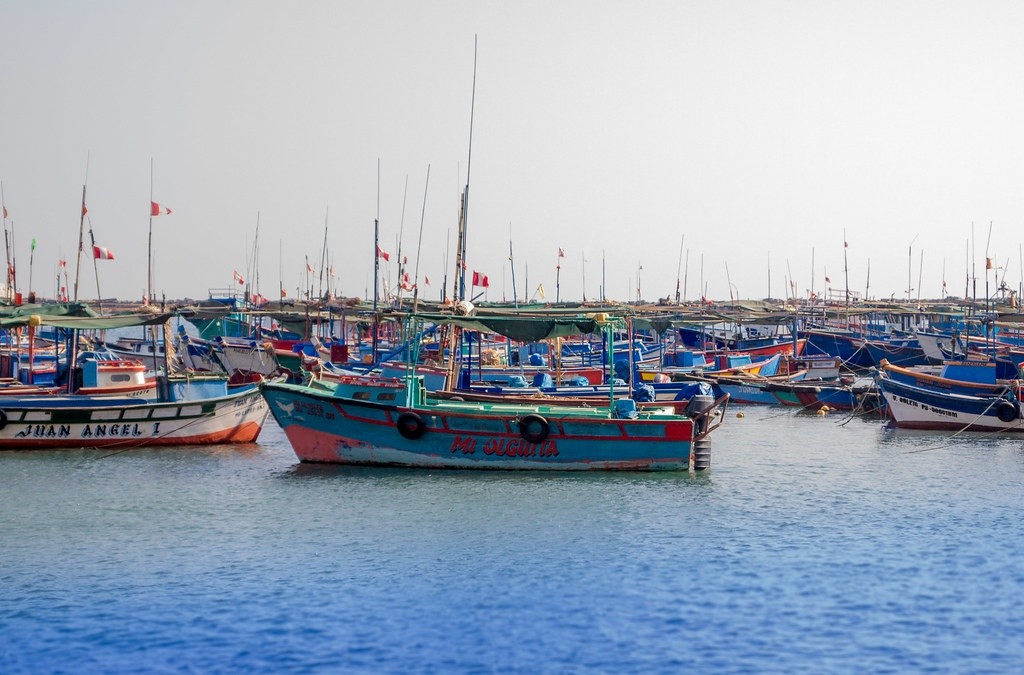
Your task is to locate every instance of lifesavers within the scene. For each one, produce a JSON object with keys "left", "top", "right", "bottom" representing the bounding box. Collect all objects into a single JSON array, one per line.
[
  {"left": 998, "top": 401, "right": 1016, "bottom": 422},
  {"left": 519, "top": 414, "right": 549, "bottom": 444},
  {"left": 396, "top": 411, "right": 425, "bottom": 439}
]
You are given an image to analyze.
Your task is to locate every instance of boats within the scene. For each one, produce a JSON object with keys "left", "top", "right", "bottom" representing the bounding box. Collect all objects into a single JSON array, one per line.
[
  {"left": 256, "top": 299, "right": 730, "bottom": 470},
  {"left": 0, "top": 304, "right": 290, "bottom": 448},
  {"left": 0, "top": 33, "right": 1023, "bottom": 433}
]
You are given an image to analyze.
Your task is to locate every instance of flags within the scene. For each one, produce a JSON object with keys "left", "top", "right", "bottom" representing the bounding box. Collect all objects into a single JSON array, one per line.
[
  {"left": 404, "top": 274, "right": 411, "bottom": 283},
  {"left": 559, "top": 248, "right": 564, "bottom": 257},
  {"left": 3, "top": 208, "right": 8, "bottom": 218},
  {"left": 282, "top": 290, "right": 286, "bottom": 297},
  {"left": 307, "top": 264, "right": 316, "bottom": 274},
  {"left": 539, "top": 286, "right": 544, "bottom": 299},
  {"left": 94, "top": 246, "right": 113, "bottom": 259},
  {"left": 234, "top": 272, "right": 244, "bottom": 285},
  {"left": 425, "top": 276, "right": 431, "bottom": 286},
  {"left": 402, "top": 281, "right": 414, "bottom": 292},
  {"left": 473, "top": 272, "right": 489, "bottom": 287},
  {"left": 378, "top": 247, "right": 389, "bottom": 261},
  {"left": 151, "top": 202, "right": 171, "bottom": 216},
  {"left": 10, "top": 266, "right": 15, "bottom": 280}
]
